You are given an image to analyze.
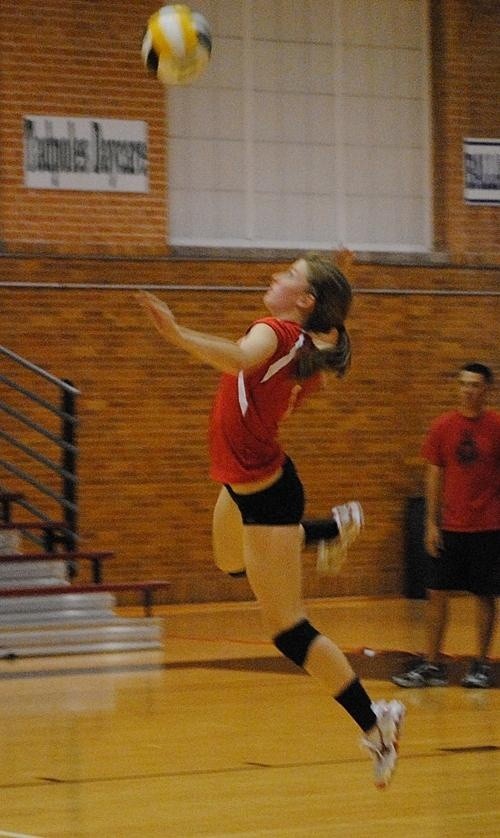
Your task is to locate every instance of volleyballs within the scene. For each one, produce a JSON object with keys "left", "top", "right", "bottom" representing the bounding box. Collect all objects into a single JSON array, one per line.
[{"left": 142, "top": 5, "right": 210, "bottom": 84}]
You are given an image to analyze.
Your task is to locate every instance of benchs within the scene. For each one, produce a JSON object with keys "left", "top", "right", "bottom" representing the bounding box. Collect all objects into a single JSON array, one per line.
[{"left": 0, "top": 487, "right": 169, "bottom": 661}]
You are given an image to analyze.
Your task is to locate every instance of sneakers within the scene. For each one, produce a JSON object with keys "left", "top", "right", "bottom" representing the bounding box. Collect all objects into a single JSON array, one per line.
[
  {"left": 362, "top": 698, "right": 407, "bottom": 786},
  {"left": 315, "top": 500, "right": 365, "bottom": 575},
  {"left": 391, "top": 662, "right": 450, "bottom": 688},
  {"left": 460, "top": 654, "right": 498, "bottom": 688}
]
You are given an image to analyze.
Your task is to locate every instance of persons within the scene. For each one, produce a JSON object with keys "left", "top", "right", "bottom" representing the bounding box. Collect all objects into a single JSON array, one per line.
[
  {"left": 390, "top": 360, "right": 499, "bottom": 689},
  {"left": 128, "top": 245, "right": 405, "bottom": 789}
]
